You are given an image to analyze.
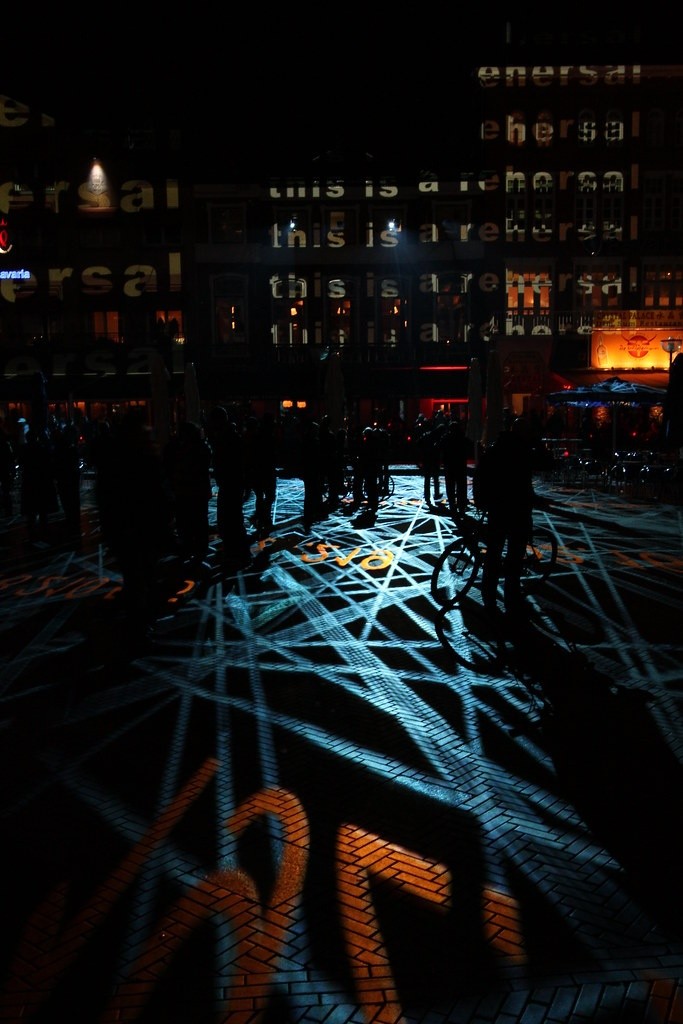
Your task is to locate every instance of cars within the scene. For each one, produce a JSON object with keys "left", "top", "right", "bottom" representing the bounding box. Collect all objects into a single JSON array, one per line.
[
  {"left": 611, "top": 451, "right": 676, "bottom": 483},
  {"left": 532, "top": 438, "right": 595, "bottom": 471}
]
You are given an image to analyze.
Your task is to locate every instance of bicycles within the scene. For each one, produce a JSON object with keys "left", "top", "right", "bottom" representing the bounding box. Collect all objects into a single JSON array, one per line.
[
  {"left": 322, "top": 454, "right": 394, "bottom": 502},
  {"left": 431, "top": 497, "right": 557, "bottom": 605}
]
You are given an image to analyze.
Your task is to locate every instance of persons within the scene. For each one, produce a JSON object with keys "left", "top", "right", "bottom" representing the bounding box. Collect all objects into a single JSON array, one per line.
[{"left": 0, "top": 400, "right": 683, "bottom": 618}]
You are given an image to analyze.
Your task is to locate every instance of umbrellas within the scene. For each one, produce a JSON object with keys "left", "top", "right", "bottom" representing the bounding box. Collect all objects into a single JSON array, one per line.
[{"left": 549, "top": 377, "right": 667, "bottom": 457}]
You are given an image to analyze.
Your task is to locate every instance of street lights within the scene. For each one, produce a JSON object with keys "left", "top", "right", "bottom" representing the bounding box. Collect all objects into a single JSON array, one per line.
[{"left": 661, "top": 338, "right": 682, "bottom": 383}]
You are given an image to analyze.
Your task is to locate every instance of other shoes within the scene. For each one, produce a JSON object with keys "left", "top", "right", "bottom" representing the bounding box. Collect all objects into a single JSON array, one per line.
[
  {"left": 427, "top": 501, "right": 431, "bottom": 506},
  {"left": 434, "top": 494, "right": 443, "bottom": 500},
  {"left": 456, "top": 499, "right": 469, "bottom": 508},
  {"left": 450, "top": 501, "right": 457, "bottom": 511}
]
